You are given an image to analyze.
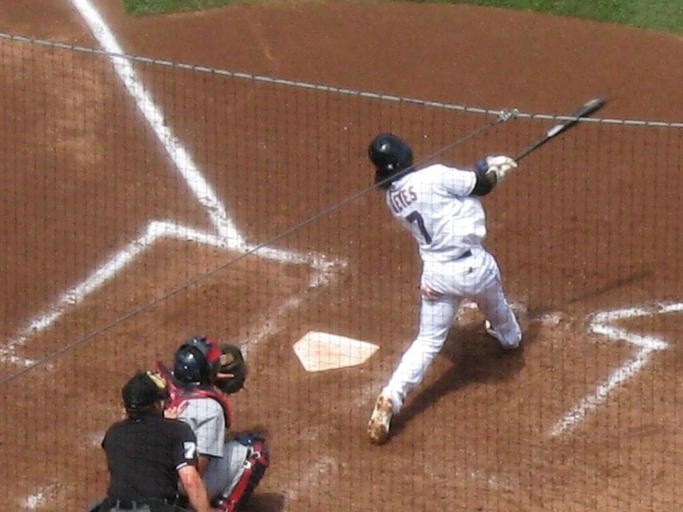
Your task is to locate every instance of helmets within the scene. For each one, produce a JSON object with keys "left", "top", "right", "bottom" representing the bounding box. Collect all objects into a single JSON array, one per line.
[
  {"left": 173, "top": 335, "right": 224, "bottom": 394},
  {"left": 367, "top": 133, "right": 416, "bottom": 174}
]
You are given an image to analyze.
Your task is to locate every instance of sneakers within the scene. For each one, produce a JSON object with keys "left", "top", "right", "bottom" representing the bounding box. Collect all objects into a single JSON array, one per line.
[
  {"left": 367, "top": 389, "right": 395, "bottom": 445},
  {"left": 486, "top": 320, "right": 499, "bottom": 339}
]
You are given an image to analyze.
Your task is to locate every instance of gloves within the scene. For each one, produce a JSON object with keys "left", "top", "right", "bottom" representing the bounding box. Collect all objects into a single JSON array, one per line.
[{"left": 485, "top": 156, "right": 518, "bottom": 182}]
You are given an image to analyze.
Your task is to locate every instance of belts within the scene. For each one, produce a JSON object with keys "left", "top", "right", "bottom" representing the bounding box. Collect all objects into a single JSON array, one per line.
[
  {"left": 108, "top": 500, "right": 168, "bottom": 510},
  {"left": 454, "top": 248, "right": 472, "bottom": 262}
]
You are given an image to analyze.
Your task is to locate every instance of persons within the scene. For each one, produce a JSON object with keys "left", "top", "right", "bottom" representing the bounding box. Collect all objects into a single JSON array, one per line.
[
  {"left": 366, "top": 132, "right": 521, "bottom": 444},
  {"left": 153, "top": 336, "right": 271, "bottom": 511},
  {"left": 82, "top": 370, "right": 224, "bottom": 512}
]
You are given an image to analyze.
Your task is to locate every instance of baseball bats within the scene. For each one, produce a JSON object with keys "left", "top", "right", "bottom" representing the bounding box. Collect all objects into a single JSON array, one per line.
[{"left": 515, "top": 99, "right": 603, "bottom": 162}]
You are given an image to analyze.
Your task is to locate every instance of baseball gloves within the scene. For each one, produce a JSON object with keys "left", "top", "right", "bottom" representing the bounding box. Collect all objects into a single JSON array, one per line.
[{"left": 209, "top": 345, "right": 246, "bottom": 394}]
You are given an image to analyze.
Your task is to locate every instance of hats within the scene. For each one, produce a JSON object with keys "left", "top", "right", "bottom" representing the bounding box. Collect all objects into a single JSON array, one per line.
[{"left": 121, "top": 370, "right": 169, "bottom": 411}]
